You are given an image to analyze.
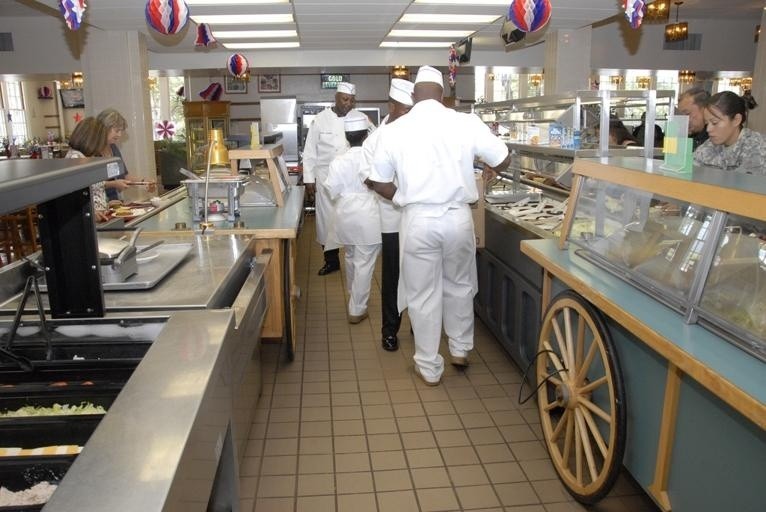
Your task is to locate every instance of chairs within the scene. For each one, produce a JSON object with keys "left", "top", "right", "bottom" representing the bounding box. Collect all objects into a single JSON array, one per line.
[{"left": 0, "top": 204, "right": 43, "bottom": 267}]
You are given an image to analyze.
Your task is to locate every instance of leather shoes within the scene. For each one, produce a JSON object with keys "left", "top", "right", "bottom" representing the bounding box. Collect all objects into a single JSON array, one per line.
[
  {"left": 318, "top": 263, "right": 340, "bottom": 276},
  {"left": 381, "top": 336, "right": 398, "bottom": 351}
]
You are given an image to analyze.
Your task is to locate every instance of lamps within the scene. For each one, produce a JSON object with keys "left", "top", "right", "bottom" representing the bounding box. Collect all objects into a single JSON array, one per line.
[
  {"left": 642, "top": 0, "right": 670, "bottom": 24},
  {"left": 200, "top": 126, "right": 230, "bottom": 227},
  {"left": 665, "top": 2, "right": 688, "bottom": 42}
]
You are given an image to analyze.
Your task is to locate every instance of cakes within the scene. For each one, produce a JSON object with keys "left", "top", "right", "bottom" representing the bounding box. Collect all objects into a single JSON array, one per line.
[{"left": 116, "top": 208, "right": 133, "bottom": 216}]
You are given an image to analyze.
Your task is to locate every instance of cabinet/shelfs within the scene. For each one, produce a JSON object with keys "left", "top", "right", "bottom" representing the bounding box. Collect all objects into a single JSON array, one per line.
[{"left": 183, "top": 101, "right": 230, "bottom": 171}]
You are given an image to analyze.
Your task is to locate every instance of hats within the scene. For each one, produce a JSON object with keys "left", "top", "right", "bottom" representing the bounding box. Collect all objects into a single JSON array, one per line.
[
  {"left": 414, "top": 65, "right": 444, "bottom": 89},
  {"left": 336, "top": 81, "right": 356, "bottom": 96},
  {"left": 388, "top": 78, "right": 415, "bottom": 107},
  {"left": 343, "top": 108, "right": 368, "bottom": 132}
]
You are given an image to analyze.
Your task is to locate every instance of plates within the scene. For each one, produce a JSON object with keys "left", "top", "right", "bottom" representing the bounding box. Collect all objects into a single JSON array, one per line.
[
  {"left": 135, "top": 253, "right": 161, "bottom": 264},
  {"left": 110, "top": 201, "right": 156, "bottom": 219}
]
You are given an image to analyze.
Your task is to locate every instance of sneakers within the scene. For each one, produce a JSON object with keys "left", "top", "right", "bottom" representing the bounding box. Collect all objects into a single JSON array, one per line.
[
  {"left": 449, "top": 357, "right": 470, "bottom": 368},
  {"left": 414, "top": 364, "right": 442, "bottom": 387},
  {"left": 348, "top": 311, "right": 369, "bottom": 325}
]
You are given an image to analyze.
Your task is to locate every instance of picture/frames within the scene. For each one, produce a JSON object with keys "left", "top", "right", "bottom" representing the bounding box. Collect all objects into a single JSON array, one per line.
[
  {"left": 224, "top": 75, "right": 248, "bottom": 94},
  {"left": 258, "top": 74, "right": 281, "bottom": 93}
]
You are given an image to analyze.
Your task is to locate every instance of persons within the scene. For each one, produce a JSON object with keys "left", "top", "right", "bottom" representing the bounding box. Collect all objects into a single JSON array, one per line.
[
  {"left": 96, "top": 108, "right": 144, "bottom": 200},
  {"left": 677, "top": 89, "right": 711, "bottom": 152},
  {"left": 63, "top": 115, "right": 123, "bottom": 224},
  {"left": 594, "top": 113, "right": 642, "bottom": 146},
  {"left": 300, "top": 81, "right": 379, "bottom": 275},
  {"left": 322, "top": 109, "right": 382, "bottom": 325},
  {"left": 358, "top": 63, "right": 512, "bottom": 387},
  {"left": 632, "top": 111, "right": 664, "bottom": 139},
  {"left": 362, "top": 77, "right": 415, "bottom": 351},
  {"left": 692, "top": 90, "right": 766, "bottom": 173}
]
